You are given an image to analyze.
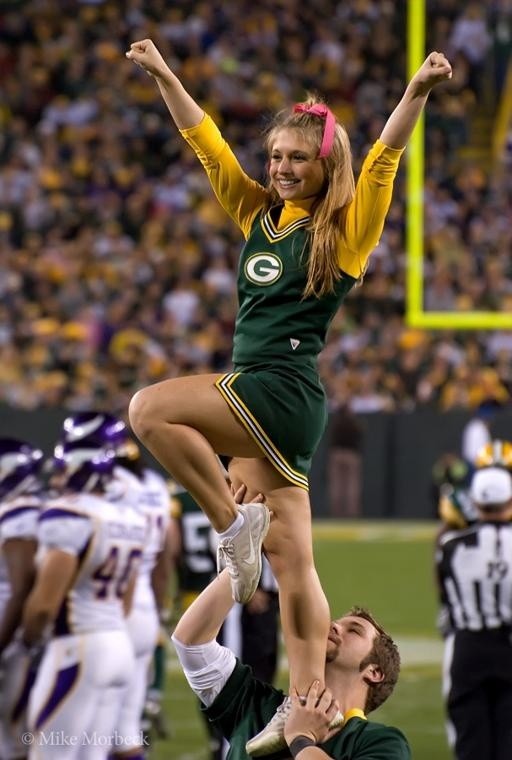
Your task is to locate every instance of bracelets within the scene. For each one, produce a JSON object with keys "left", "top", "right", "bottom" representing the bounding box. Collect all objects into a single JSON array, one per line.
[{"left": 288, "top": 734, "right": 316, "bottom": 757}]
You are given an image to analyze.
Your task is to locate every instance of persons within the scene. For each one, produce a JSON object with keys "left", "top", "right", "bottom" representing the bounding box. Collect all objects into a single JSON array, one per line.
[
  {"left": 428, "top": 417, "right": 512, "bottom": 759},
  {"left": 207, "top": 547, "right": 282, "bottom": 759},
  {"left": 165, "top": 475, "right": 220, "bottom": 615},
  {"left": 326, "top": 399, "right": 366, "bottom": 521},
  {"left": 123, "top": 36, "right": 458, "bottom": 759},
  {"left": 0, "top": 411, "right": 172, "bottom": 760},
  {"left": 166, "top": 481, "right": 417, "bottom": 760},
  {"left": 2, "top": 0, "right": 511, "bottom": 413}
]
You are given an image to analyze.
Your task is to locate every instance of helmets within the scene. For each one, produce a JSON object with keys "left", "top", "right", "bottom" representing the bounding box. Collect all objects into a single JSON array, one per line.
[{"left": 1, "top": 410, "right": 142, "bottom": 505}]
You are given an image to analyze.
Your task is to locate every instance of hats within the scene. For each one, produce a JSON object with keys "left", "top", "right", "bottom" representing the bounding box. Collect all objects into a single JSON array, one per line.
[{"left": 469, "top": 467, "right": 512, "bottom": 506}]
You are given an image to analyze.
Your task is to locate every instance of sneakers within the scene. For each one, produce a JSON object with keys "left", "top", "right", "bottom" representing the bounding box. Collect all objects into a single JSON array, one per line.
[
  {"left": 245, "top": 695, "right": 345, "bottom": 758},
  {"left": 216, "top": 502, "right": 271, "bottom": 606}
]
